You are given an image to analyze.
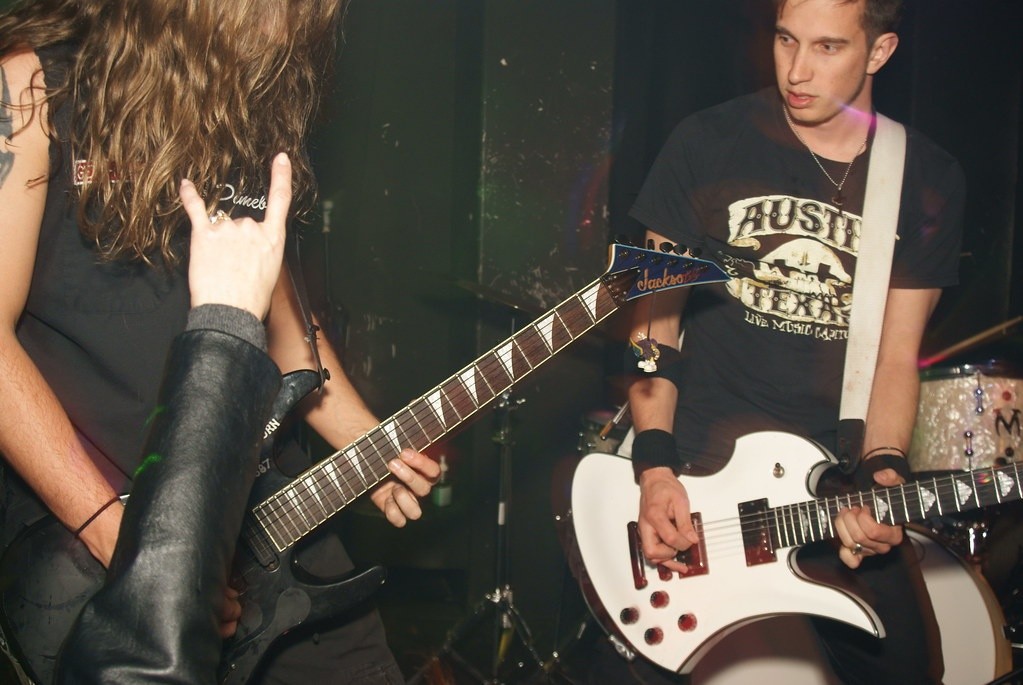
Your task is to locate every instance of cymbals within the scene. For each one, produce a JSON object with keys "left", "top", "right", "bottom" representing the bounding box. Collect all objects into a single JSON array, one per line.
[{"left": 453, "top": 279, "right": 539, "bottom": 316}]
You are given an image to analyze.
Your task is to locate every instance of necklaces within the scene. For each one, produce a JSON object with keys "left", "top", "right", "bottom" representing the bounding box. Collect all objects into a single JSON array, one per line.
[{"left": 783, "top": 103, "right": 868, "bottom": 203}]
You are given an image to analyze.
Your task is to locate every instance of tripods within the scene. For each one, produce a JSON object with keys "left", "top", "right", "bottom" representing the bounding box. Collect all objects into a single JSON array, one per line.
[{"left": 401, "top": 312, "right": 552, "bottom": 685}]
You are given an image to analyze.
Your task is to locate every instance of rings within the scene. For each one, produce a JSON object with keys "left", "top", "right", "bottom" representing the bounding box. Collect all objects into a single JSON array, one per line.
[{"left": 851, "top": 543, "right": 862, "bottom": 555}]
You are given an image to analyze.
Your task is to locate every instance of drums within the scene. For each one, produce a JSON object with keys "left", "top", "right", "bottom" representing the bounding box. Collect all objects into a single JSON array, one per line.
[
  {"left": 688, "top": 522, "right": 1016, "bottom": 685},
  {"left": 904, "top": 358, "right": 1023, "bottom": 480}
]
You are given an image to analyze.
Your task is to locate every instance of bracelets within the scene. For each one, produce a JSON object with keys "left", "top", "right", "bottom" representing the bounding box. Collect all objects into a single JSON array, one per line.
[
  {"left": 862, "top": 447, "right": 908, "bottom": 456},
  {"left": 631, "top": 428, "right": 681, "bottom": 483},
  {"left": 73, "top": 496, "right": 121, "bottom": 536}
]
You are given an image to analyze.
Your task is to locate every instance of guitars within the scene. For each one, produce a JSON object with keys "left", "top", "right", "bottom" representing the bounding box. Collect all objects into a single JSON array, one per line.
[
  {"left": 559, "top": 428, "right": 1023, "bottom": 677},
  {"left": 0, "top": 230, "right": 735, "bottom": 685}
]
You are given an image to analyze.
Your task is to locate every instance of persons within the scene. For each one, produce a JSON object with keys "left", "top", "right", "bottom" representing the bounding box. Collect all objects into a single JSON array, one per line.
[
  {"left": 0, "top": 0, "right": 441, "bottom": 685},
  {"left": 622, "top": 0, "right": 967, "bottom": 570}
]
